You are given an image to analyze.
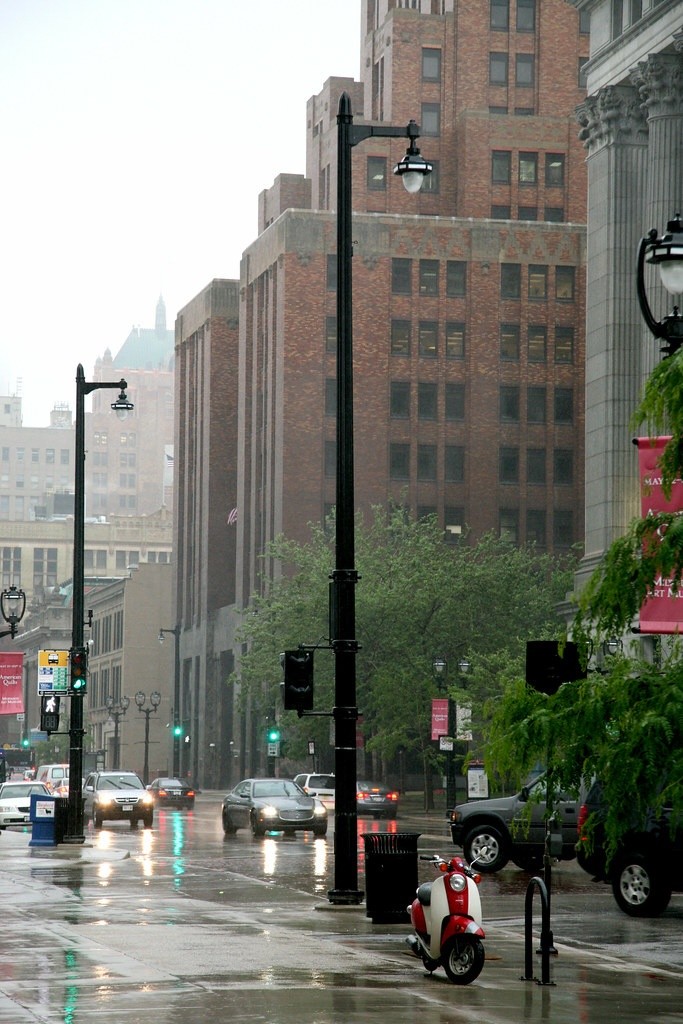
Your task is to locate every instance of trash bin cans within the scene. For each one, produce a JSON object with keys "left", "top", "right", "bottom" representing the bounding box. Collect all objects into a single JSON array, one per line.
[
  {"left": 359, "top": 831, "right": 424, "bottom": 924},
  {"left": 52, "top": 798, "right": 88, "bottom": 844}
]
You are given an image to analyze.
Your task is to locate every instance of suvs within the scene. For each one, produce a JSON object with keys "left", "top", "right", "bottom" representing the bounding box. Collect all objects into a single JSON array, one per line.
[
  {"left": 445, "top": 759, "right": 600, "bottom": 873},
  {"left": 573, "top": 760, "right": 683, "bottom": 916}
]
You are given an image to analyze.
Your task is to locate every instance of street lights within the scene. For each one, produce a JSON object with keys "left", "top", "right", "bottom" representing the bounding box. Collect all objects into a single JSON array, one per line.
[
  {"left": 134, "top": 690, "right": 162, "bottom": 787},
  {"left": 106, "top": 694, "right": 129, "bottom": 772},
  {"left": 251, "top": 606, "right": 276, "bottom": 778},
  {"left": 158, "top": 625, "right": 181, "bottom": 777},
  {"left": 325, "top": 90, "right": 435, "bottom": 904},
  {"left": 62, "top": 363, "right": 135, "bottom": 846},
  {"left": 432, "top": 657, "right": 473, "bottom": 818}
]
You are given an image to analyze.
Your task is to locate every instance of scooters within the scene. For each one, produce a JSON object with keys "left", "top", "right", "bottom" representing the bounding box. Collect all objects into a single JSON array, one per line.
[{"left": 404, "top": 847, "right": 497, "bottom": 987}]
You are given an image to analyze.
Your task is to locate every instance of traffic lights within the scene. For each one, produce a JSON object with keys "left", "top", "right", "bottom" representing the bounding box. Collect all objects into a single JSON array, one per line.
[
  {"left": 69, "top": 650, "right": 88, "bottom": 693},
  {"left": 271, "top": 733, "right": 277, "bottom": 740},
  {"left": 40, "top": 696, "right": 60, "bottom": 732},
  {"left": 24, "top": 741, "right": 28, "bottom": 745},
  {"left": 175, "top": 728, "right": 180, "bottom": 735}
]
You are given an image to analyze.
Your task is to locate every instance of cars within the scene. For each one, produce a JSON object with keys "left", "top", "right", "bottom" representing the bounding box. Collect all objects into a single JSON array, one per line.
[
  {"left": 0, "top": 765, "right": 155, "bottom": 828},
  {"left": 221, "top": 777, "right": 328, "bottom": 838},
  {"left": 356, "top": 779, "right": 402, "bottom": 818},
  {"left": 291, "top": 771, "right": 335, "bottom": 812},
  {"left": 146, "top": 777, "right": 195, "bottom": 811}
]
